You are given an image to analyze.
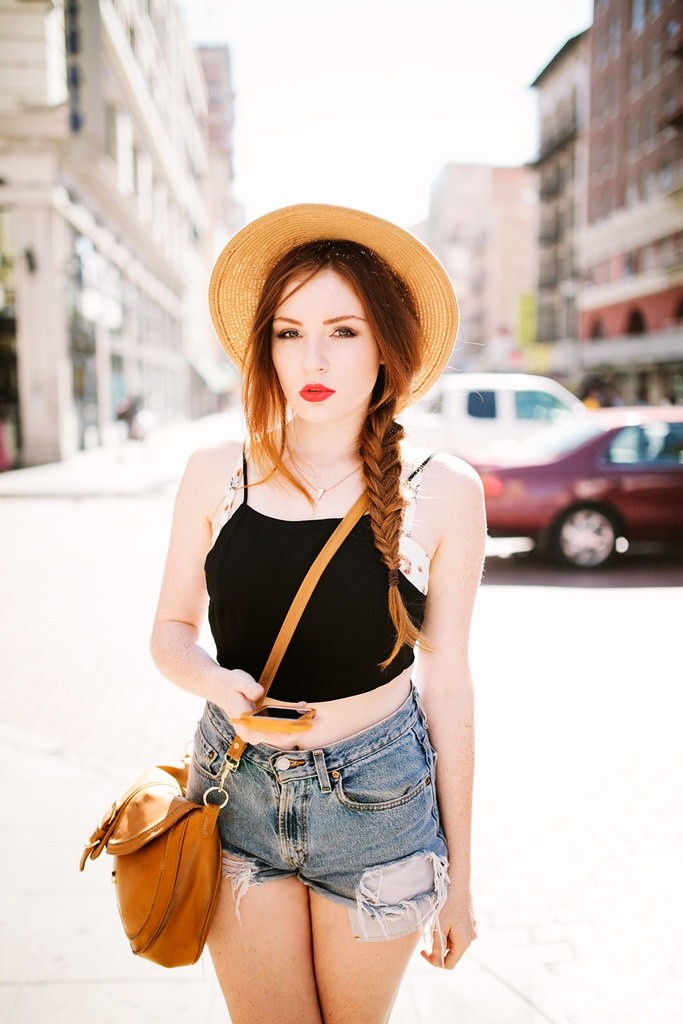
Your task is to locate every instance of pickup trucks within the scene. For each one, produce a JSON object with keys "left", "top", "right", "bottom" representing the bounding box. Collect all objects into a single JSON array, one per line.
[{"left": 389, "top": 375, "right": 603, "bottom": 466}]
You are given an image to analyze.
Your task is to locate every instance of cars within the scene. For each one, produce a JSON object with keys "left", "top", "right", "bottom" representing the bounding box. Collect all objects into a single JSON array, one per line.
[{"left": 454, "top": 403, "right": 683, "bottom": 573}]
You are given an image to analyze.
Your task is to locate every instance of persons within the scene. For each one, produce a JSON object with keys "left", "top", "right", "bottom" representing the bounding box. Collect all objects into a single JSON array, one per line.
[{"left": 151, "top": 202, "right": 487, "bottom": 1024}]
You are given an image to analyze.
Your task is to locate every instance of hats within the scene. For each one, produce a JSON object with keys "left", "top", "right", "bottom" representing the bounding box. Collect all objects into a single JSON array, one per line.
[{"left": 203, "top": 199, "right": 464, "bottom": 414}]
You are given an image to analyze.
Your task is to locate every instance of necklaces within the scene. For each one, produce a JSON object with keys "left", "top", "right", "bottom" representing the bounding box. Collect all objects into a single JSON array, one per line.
[{"left": 286, "top": 445, "right": 362, "bottom": 501}]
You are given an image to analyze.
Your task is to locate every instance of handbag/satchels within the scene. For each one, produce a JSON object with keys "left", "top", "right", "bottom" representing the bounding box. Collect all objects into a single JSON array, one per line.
[{"left": 77, "top": 749, "right": 231, "bottom": 972}]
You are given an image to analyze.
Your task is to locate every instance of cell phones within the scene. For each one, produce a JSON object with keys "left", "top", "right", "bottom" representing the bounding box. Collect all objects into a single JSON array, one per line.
[{"left": 231, "top": 705, "right": 317, "bottom": 735}]
